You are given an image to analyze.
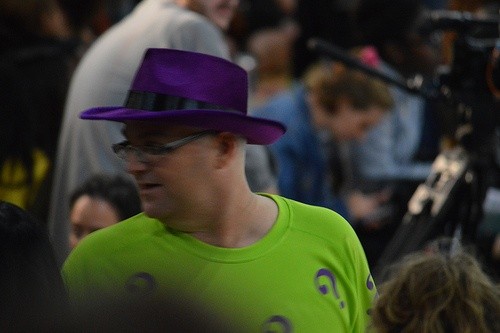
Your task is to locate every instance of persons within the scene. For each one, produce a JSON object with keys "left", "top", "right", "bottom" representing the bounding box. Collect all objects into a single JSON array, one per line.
[
  {"left": 0, "top": 0, "right": 500, "bottom": 333},
  {"left": 60, "top": 47, "right": 380, "bottom": 333}
]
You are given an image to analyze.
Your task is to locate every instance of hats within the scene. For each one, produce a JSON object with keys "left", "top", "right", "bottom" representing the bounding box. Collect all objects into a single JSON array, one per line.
[{"left": 77, "top": 48, "right": 287, "bottom": 145}]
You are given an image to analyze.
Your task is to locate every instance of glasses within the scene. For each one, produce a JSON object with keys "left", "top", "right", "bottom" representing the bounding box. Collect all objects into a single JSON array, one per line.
[{"left": 111, "top": 128, "right": 222, "bottom": 166}]
